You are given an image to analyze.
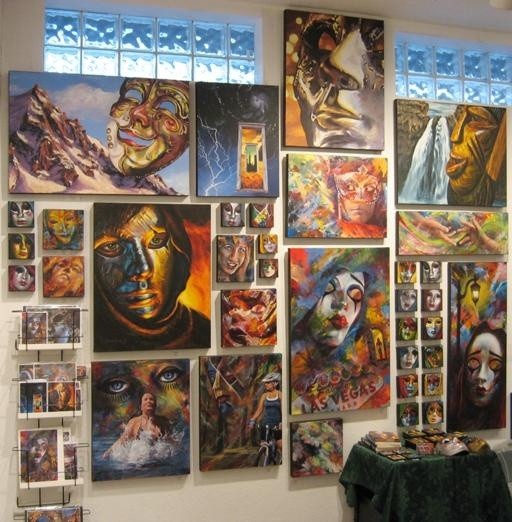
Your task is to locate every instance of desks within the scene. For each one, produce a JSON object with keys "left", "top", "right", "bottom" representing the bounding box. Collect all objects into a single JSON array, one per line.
[{"left": 350, "top": 430, "right": 500, "bottom": 522}]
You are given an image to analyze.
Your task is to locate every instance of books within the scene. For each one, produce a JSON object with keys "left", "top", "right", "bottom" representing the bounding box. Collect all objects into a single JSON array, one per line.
[{"left": 358, "top": 427, "right": 401, "bottom": 452}]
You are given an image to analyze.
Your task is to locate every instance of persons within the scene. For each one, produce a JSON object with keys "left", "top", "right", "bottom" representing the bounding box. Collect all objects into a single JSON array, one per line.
[
  {"left": 94, "top": 203, "right": 212, "bottom": 349},
  {"left": 27, "top": 317, "right": 41, "bottom": 336},
  {"left": 45, "top": 206, "right": 85, "bottom": 250},
  {"left": 290, "top": 12, "right": 384, "bottom": 151},
  {"left": 306, "top": 268, "right": 366, "bottom": 349},
  {"left": 7, "top": 199, "right": 36, "bottom": 228},
  {"left": 103, "top": 390, "right": 171, "bottom": 460},
  {"left": 446, "top": 104, "right": 507, "bottom": 205},
  {"left": 44, "top": 256, "right": 85, "bottom": 297},
  {"left": 326, "top": 156, "right": 388, "bottom": 223},
  {"left": 215, "top": 389, "right": 235, "bottom": 419},
  {"left": 8, "top": 233, "right": 35, "bottom": 261},
  {"left": 248, "top": 373, "right": 282, "bottom": 459},
  {"left": 9, "top": 264, "right": 35, "bottom": 291},
  {"left": 214, "top": 197, "right": 282, "bottom": 349},
  {"left": 91, "top": 362, "right": 189, "bottom": 436},
  {"left": 21, "top": 431, "right": 58, "bottom": 484},
  {"left": 397, "top": 212, "right": 504, "bottom": 434}
]
